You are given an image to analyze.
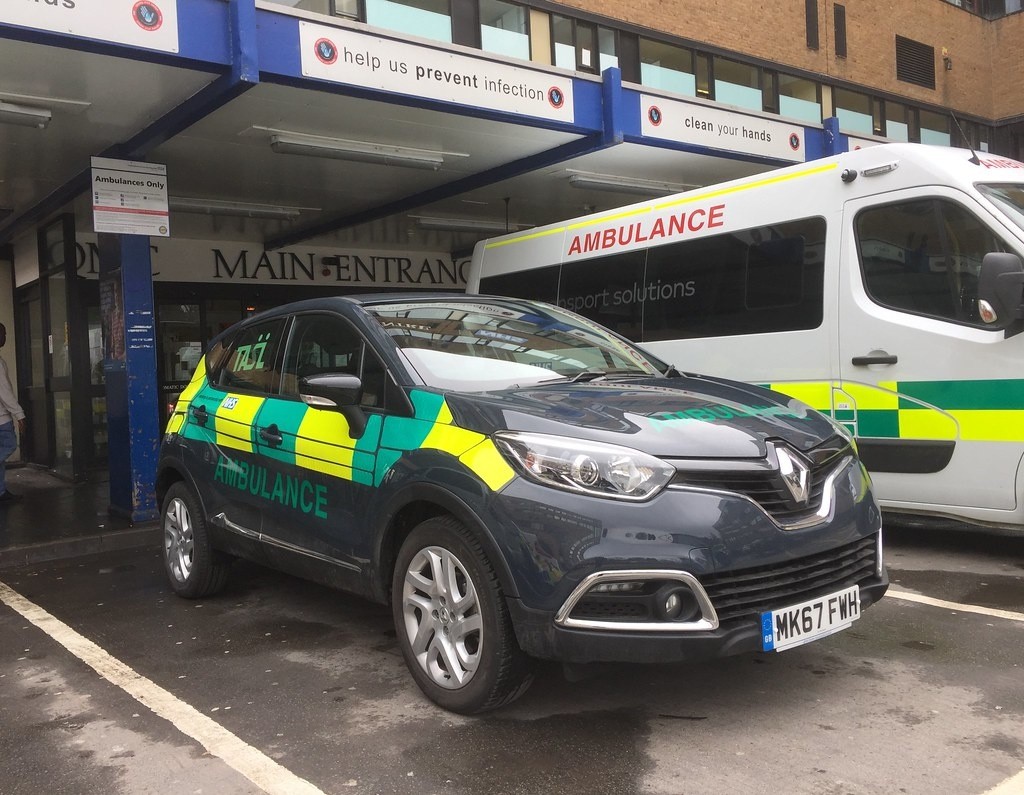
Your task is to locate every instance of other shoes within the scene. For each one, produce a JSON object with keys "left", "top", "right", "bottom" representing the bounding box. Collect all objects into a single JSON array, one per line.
[{"left": 0, "top": 490, "right": 24, "bottom": 505}]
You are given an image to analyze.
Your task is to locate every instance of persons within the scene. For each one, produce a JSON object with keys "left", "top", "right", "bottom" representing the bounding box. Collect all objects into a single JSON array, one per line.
[
  {"left": 0, "top": 322, "right": 26, "bottom": 503},
  {"left": 921, "top": 269, "right": 978, "bottom": 321}
]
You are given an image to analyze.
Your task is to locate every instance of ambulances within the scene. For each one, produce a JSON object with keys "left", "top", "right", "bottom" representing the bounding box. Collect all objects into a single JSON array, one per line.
[
  {"left": 465, "top": 113, "right": 1024, "bottom": 538},
  {"left": 155, "top": 293, "right": 890, "bottom": 717}
]
